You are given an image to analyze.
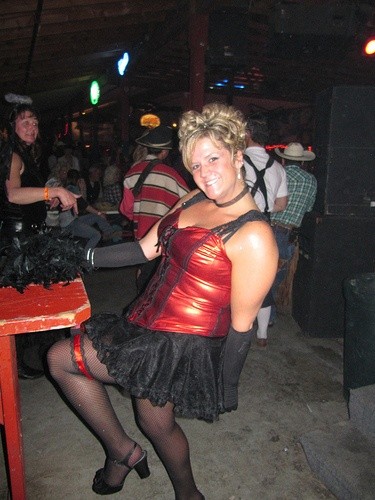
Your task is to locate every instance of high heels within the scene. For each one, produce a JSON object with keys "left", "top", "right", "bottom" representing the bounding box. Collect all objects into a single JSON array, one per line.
[{"left": 92, "top": 443, "right": 151, "bottom": 495}]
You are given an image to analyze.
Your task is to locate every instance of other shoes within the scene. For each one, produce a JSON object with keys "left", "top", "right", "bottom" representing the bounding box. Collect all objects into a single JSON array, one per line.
[
  {"left": 18, "top": 364, "right": 44, "bottom": 379},
  {"left": 254, "top": 333, "right": 267, "bottom": 351}
]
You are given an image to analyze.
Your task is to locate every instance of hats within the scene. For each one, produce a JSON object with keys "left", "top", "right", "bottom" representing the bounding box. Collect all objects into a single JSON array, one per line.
[
  {"left": 135, "top": 125, "right": 172, "bottom": 150},
  {"left": 274, "top": 142, "right": 316, "bottom": 161}
]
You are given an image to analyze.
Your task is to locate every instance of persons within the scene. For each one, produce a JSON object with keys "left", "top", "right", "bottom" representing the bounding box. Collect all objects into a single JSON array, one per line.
[
  {"left": 0, "top": 105, "right": 83, "bottom": 379},
  {"left": 119, "top": 126, "right": 191, "bottom": 296},
  {"left": 46, "top": 103, "right": 278, "bottom": 500},
  {"left": 39, "top": 137, "right": 134, "bottom": 249},
  {"left": 241, "top": 112, "right": 289, "bottom": 346},
  {"left": 269, "top": 142, "right": 317, "bottom": 327}
]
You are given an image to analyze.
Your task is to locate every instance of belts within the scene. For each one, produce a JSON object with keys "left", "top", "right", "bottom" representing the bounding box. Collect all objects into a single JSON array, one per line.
[{"left": 276, "top": 222, "right": 294, "bottom": 231}]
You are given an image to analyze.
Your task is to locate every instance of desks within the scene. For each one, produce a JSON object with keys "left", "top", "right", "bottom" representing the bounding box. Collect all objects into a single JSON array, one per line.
[{"left": 0, "top": 272, "right": 91, "bottom": 500}]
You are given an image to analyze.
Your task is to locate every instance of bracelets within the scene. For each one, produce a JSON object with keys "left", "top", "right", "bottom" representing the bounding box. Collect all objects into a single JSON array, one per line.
[
  {"left": 92, "top": 249, "right": 98, "bottom": 271},
  {"left": 45, "top": 187, "right": 48, "bottom": 200},
  {"left": 86, "top": 248, "right": 91, "bottom": 263},
  {"left": 99, "top": 213, "right": 103, "bottom": 217}
]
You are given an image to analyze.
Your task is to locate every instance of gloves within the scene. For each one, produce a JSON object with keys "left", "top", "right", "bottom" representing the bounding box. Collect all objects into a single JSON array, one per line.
[
  {"left": 221, "top": 327, "right": 254, "bottom": 414},
  {"left": 83, "top": 241, "right": 149, "bottom": 269}
]
